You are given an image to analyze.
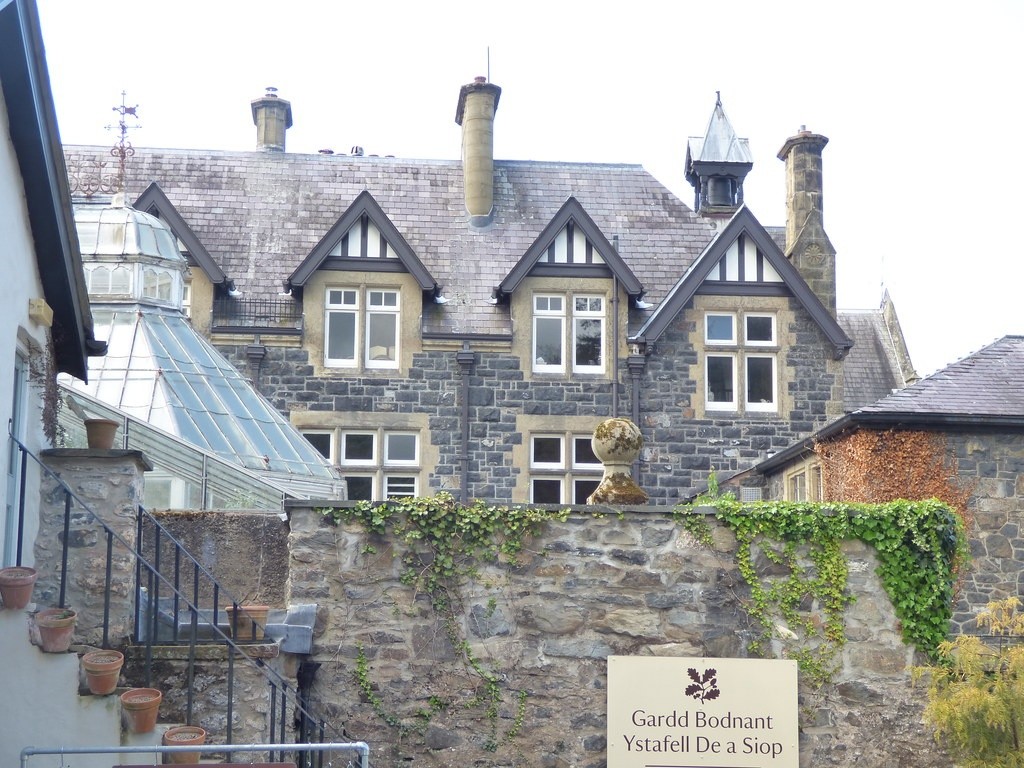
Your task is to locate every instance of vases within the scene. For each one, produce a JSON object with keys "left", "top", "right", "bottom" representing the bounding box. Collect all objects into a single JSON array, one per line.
[
  {"left": 225, "top": 605, "right": 271, "bottom": 641},
  {"left": 33, "top": 608, "right": 77, "bottom": 652},
  {"left": 120, "top": 687, "right": 162, "bottom": 734},
  {"left": 163, "top": 726, "right": 207, "bottom": 765},
  {"left": 1, "top": 566, "right": 37, "bottom": 608},
  {"left": 84, "top": 418, "right": 120, "bottom": 449},
  {"left": 80, "top": 649, "right": 126, "bottom": 695}
]
